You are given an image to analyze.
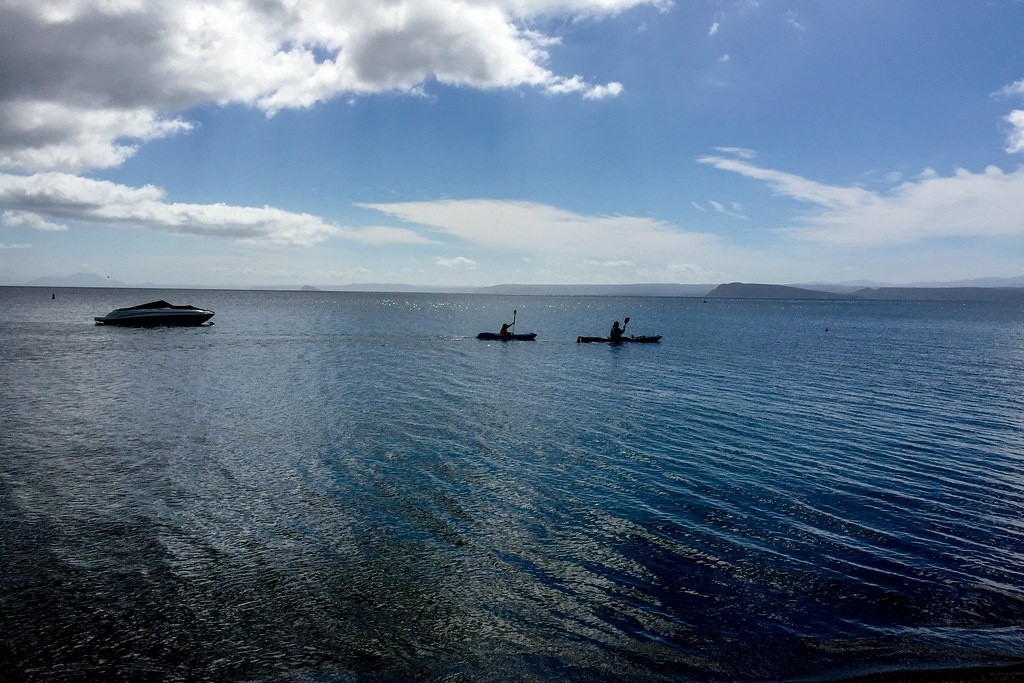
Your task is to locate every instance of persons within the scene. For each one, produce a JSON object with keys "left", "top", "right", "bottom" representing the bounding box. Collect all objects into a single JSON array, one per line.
[
  {"left": 499, "top": 321, "right": 515, "bottom": 337},
  {"left": 610, "top": 321, "right": 626, "bottom": 339}
]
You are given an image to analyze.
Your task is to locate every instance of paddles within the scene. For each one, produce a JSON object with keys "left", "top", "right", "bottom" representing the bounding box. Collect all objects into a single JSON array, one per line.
[
  {"left": 513, "top": 309, "right": 517, "bottom": 333},
  {"left": 621, "top": 318, "right": 630, "bottom": 335}
]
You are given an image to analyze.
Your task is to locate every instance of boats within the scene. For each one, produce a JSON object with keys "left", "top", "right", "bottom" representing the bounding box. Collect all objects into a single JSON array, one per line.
[
  {"left": 577, "top": 335, "right": 663, "bottom": 343},
  {"left": 94, "top": 300, "right": 216, "bottom": 326},
  {"left": 476, "top": 332, "right": 536, "bottom": 342}
]
